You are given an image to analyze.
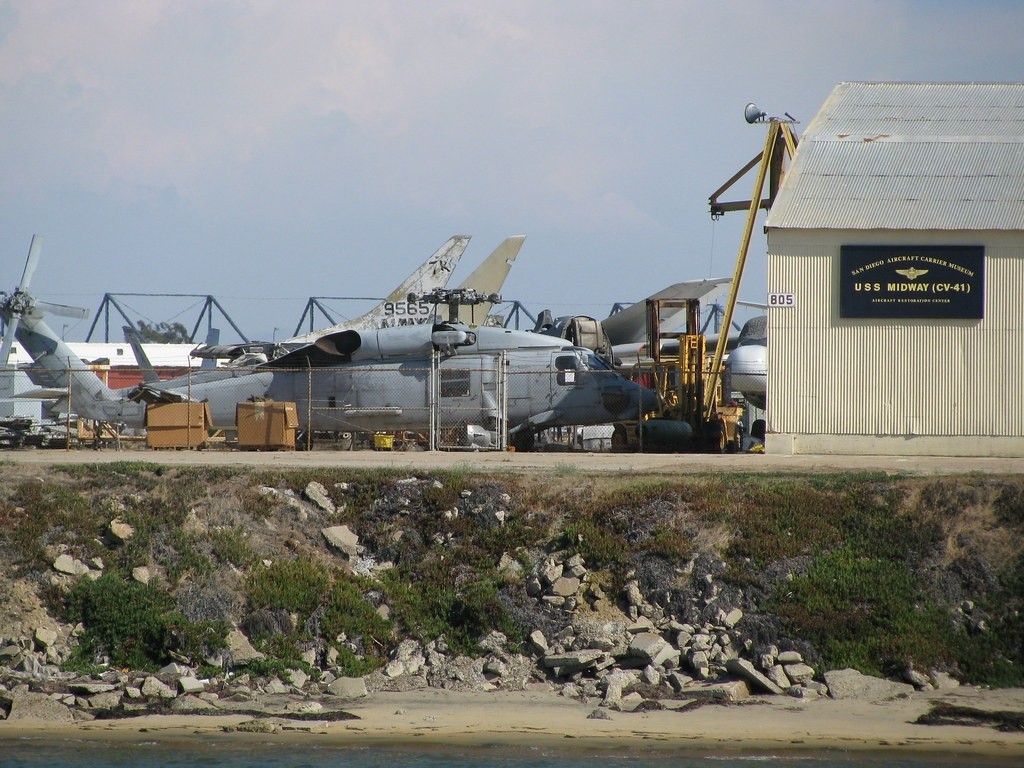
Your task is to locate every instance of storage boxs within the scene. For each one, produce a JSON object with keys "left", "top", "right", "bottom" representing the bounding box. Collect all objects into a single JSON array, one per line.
[
  {"left": 373, "top": 434, "right": 394, "bottom": 451},
  {"left": 235, "top": 402, "right": 298, "bottom": 450},
  {"left": 142, "top": 400, "right": 213, "bottom": 447}
]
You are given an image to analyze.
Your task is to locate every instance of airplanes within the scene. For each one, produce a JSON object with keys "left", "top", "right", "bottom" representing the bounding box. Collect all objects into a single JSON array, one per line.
[{"left": 0, "top": 227, "right": 773, "bottom": 461}]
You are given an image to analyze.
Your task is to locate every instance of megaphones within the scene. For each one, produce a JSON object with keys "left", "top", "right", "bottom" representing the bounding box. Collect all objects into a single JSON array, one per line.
[{"left": 744, "top": 102, "right": 768, "bottom": 124}]
